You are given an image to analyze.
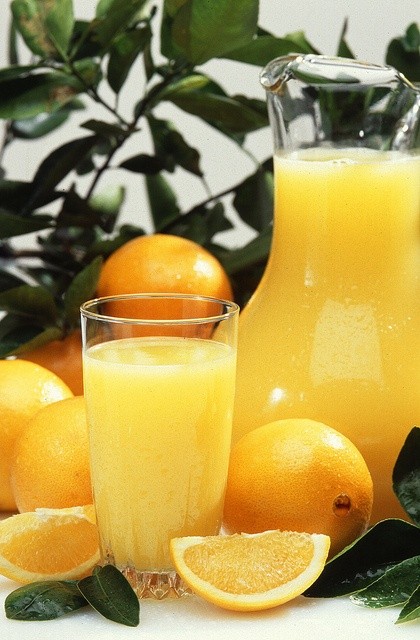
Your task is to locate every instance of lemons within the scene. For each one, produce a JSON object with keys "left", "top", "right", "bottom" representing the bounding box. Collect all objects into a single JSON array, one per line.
[
  {"left": 0, "top": 501, "right": 104, "bottom": 587},
  {"left": 94, "top": 233, "right": 234, "bottom": 340},
  {"left": 14, "top": 333, "right": 101, "bottom": 396},
  {"left": 170, "top": 529, "right": 332, "bottom": 612},
  {"left": 219, "top": 417, "right": 373, "bottom": 562},
  {"left": 0, "top": 359, "right": 75, "bottom": 512},
  {"left": 9, "top": 393, "right": 94, "bottom": 516}
]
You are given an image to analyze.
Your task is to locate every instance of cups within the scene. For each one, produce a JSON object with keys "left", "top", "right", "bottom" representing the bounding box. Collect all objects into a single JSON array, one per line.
[{"left": 80, "top": 293, "right": 242, "bottom": 601}]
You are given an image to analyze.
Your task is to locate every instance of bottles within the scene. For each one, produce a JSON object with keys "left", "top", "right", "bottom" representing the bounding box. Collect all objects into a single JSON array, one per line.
[{"left": 244, "top": 55, "right": 420, "bottom": 552}]
